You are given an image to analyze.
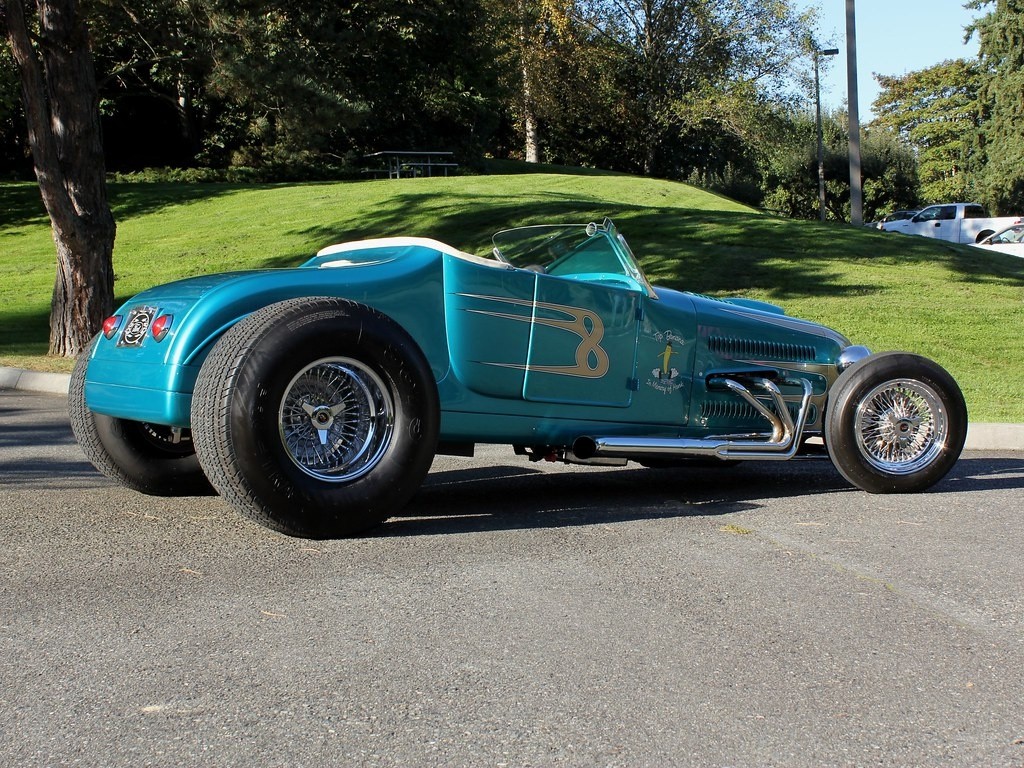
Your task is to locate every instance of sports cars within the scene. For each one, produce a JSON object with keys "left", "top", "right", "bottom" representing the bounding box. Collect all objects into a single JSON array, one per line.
[{"left": 67, "top": 213, "right": 968, "bottom": 539}]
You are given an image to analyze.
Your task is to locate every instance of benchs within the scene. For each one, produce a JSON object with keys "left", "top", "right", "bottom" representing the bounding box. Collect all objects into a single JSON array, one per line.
[
  {"left": 361, "top": 167, "right": 417, "bottom": 179},
  {"left": 402, "top": 162, "right": 460, "bottom": 178}
]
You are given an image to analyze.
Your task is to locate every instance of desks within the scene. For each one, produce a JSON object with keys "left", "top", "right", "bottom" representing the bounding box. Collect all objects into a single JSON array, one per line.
[{"left": 364, "top": 150, "right": 454, "bottom": 179}]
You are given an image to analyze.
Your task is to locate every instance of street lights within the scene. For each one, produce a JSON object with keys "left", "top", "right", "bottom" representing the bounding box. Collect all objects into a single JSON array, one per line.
[{"left": 809, "top": 49, "right": 839, "bottom": 219}]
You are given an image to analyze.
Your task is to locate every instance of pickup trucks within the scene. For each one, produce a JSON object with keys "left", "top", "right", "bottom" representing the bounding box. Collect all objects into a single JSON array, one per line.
[{"left": 866, "top": 202, "right": 1024, "bottom": 261}]
